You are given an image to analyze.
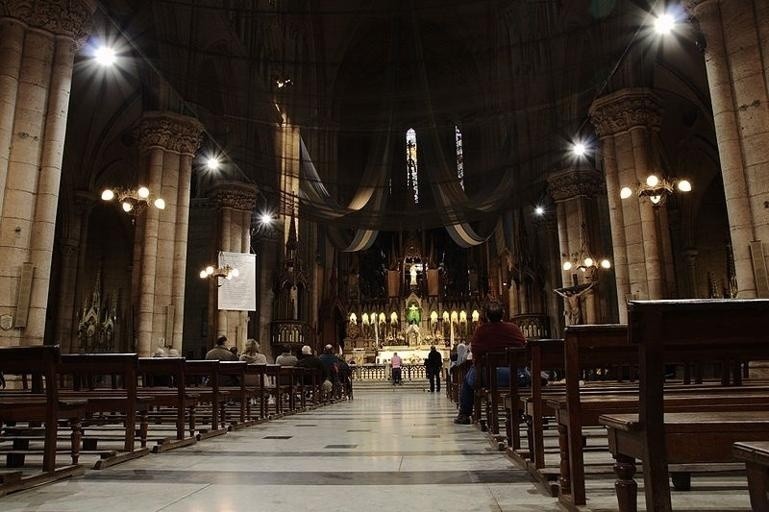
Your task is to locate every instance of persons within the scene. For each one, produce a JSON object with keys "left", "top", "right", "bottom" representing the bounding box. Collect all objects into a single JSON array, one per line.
[
  {"left": 151, "top": 335, "right": 353, "bottom": 407},
  {"left": 427, "top": 345, "right": 442, "bottom": 392},
  {"left": 448, "top": 302, "right": 606, "bottom": 425},
  {"left": 391, "top": 353, "right": 402, "bottom": 385},
  {"left": 552, "top": 281, "right": 596, "bottom": 328},
  {"left": 409, "top": 262, "right": 417, "bottom": 283}
]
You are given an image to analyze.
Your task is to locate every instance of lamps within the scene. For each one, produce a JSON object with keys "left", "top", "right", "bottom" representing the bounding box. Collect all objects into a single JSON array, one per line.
[
  {"left": 562, "top": 182, "right": 612, "bottom": 273},
  {"left": 619, "top": 40, "right": 693, "bottom": 208},
  {"left": 199, "top": 178, "right": 239, "bottom": 288},
  {"left": 100, "top": 132, "right": 166, "bottom": 217}
]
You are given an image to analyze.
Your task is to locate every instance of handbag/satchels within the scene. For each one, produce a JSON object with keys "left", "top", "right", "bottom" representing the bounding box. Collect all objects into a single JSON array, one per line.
[
  {"left": 277, "top": 353, "right": 297, "bottom": 366},
  {"left": 507, "top": 370, "right": 529, "bottom": 386}
]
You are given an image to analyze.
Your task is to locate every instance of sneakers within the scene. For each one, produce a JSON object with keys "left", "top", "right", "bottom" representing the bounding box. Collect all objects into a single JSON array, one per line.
[{"left": 454, "top": 414, "right": 470, "bottom": 424}]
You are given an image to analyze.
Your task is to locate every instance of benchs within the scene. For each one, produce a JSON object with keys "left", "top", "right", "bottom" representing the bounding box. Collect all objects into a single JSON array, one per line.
[
  {"left": 220, "top": 359, "right": 354, "bottom": 434},
  {"left": 545, "top": 323, "right": 628, "bottom": 511},
  {"left": 61, "top": 351, "right": 153, "bottom": 470},
  {"left": 519, "top": 336, "right": 564, "bottom": 499},
  {"left": 597, "top": 297, "right": 768, "bottom": 511},
  {"left": 186, "top": 359, "right": 227, "bottom": 441},
  {"left": 445, "top": 347, "right": 506, "bottom": 451},
  {"left": 504, "top": 345, "right": 529, "bottom": 470},
  {"left": 1, "top": 343, "right": 87, "bottom": 497},
  {"left": 138, "top": 357, "right": 200, "bottom": 452}
]
